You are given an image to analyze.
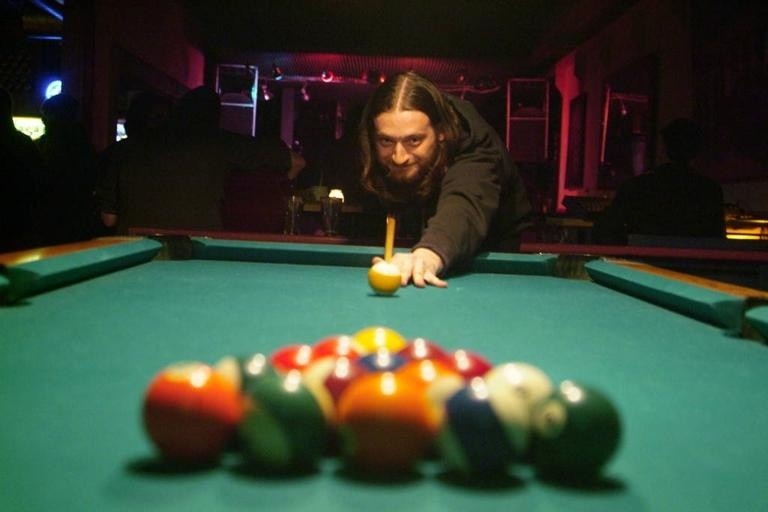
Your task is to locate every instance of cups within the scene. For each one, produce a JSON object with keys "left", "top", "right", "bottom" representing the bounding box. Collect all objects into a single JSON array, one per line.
[
  {"left": 282, "top": 196, "right": 306, "bottom": 237},
  {"left": 319, "top": 197, "right": 345, "bottom": 238},
  {"left": 310, "top": 186, "right": 329, "bottom": 202}
]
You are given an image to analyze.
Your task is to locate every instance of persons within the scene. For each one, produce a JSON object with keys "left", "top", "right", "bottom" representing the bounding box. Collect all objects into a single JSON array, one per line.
[
  {"left": 32, "top": 91, "right": 110, "bottom": 239},
  {"left": 1, "top": 87, "right": 43, "bottom": 255},
  {"left": 354, "top": 67, "right": 551, "bottom": 291},
  {"left": 588, "top": 133, "right": 726, "bottom": 249},
  {"left": 92, "top": 87, "right": 293, "bottom": 234},
  {"left": 123, "top": 91, "right": 174, "bottom": 139}
]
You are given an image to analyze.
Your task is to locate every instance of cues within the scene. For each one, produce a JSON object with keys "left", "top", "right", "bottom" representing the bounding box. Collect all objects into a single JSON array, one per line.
[{"left": 384, "top": 207, "right": 396, "bottom": 259}]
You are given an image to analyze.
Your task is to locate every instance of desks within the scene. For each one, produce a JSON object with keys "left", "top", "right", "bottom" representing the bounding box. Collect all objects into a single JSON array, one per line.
[{"left": 0, "top": 226, "right": 768, "bottom": 512}]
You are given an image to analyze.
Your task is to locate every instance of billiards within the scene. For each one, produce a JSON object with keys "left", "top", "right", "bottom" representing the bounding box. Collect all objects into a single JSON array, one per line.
[{"left": 142, "top": 327, "right": 620, "bottom": 481}]
